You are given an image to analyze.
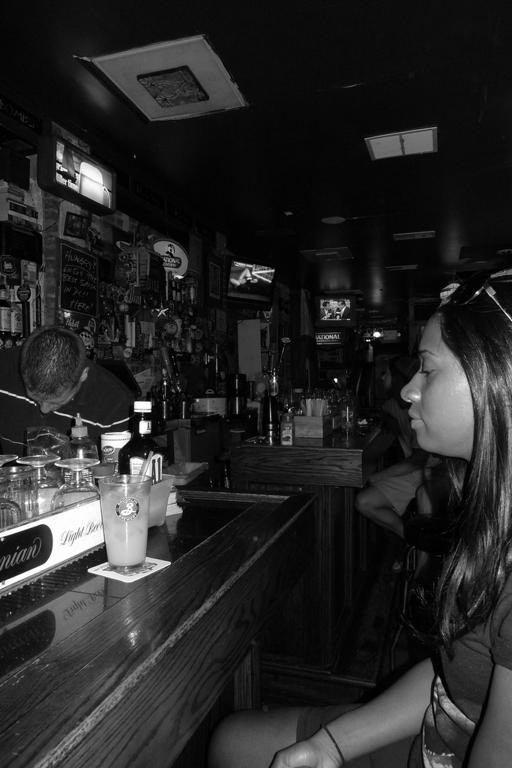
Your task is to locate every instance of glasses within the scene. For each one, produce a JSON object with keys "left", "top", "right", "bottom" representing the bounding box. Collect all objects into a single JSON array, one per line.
[{"left": 439, "top": 271, "right": 512, "bottom": 324}]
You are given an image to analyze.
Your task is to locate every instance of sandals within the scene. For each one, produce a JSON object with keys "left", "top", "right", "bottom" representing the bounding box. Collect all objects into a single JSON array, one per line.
[{"left": 391, "top": 547, "right": 408, "bottom": 573}]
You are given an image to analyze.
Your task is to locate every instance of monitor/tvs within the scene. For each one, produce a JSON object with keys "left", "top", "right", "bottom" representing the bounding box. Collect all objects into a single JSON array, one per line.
[
  {"left": 38, "top": 133, "right": 118, "bottom": 216},
  {"left": 222, "top": 255, "right": 280, "bottom": 311},
  {"left": 311, "top": 288, "right": 365, "bottom": 327}
]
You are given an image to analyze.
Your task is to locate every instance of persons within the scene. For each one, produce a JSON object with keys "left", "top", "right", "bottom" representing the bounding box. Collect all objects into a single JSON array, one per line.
[
  {"left": 0, "top": 325, "right": 134, "bottom": 474},
  {"left": 206, "top": 268, "right": 511, "bottom": 768}
]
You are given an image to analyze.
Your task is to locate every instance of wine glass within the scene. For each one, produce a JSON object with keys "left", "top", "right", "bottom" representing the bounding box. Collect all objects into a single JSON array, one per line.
[{"left": 0, "top": 454, "right": 102, "bottom": 533}]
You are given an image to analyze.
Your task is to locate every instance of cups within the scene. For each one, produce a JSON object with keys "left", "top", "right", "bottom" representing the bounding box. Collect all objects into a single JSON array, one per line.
[{"left": 95, "top": 473, "right": 153, "bottom": 575}]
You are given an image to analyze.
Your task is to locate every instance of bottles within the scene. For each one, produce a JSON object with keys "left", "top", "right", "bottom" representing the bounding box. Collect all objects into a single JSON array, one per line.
[
  {"left": 339, "top": 403, "right": 354, "bottom": 435},
  {"left": 0, "top": 281, "right": 24, "bottom": 340},
  {"left": 280, "top": 414, "right": 296, "bottom": 447},
  {"left": 68, "top": 400, "right": 164, "bottom": 480}
]
